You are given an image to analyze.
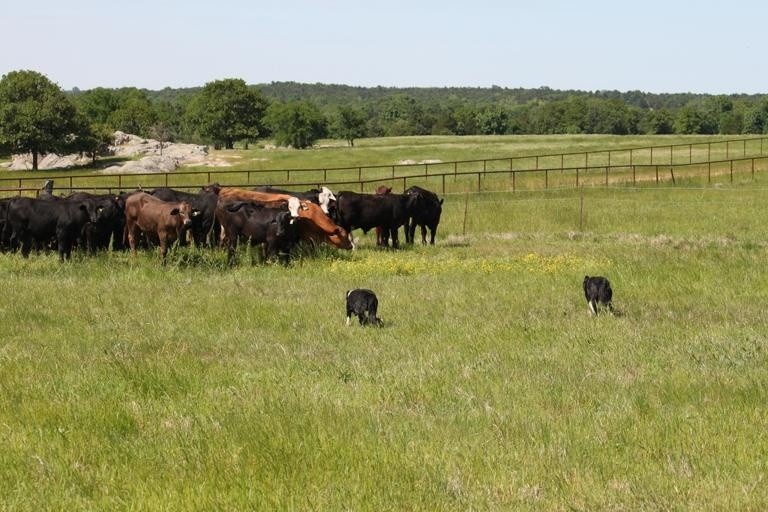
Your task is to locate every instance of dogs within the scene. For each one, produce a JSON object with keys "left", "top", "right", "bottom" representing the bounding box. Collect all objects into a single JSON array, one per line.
[
  {"left": 345, "top": 287, "right": 384, "bottom": 329},
  {"left": 582, "top": 275, "right": 619, "bottom": 318}
]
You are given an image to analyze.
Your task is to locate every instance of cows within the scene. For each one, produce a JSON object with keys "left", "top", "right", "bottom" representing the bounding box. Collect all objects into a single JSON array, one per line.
[{"left": 1, "top": 181, "right": 446, "bottom": 268}]
